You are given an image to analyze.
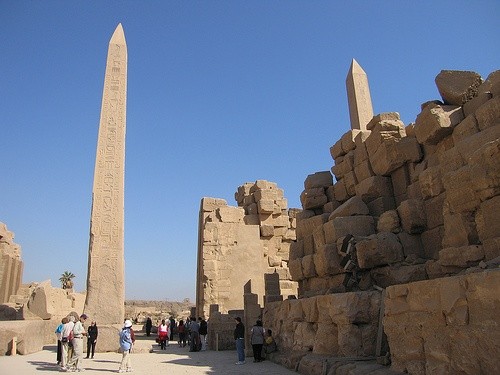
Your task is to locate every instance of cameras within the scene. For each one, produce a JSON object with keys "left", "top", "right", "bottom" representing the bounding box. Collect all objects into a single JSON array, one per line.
[{"left": 82, "top": 333, "right": 90, "bottom": 338}]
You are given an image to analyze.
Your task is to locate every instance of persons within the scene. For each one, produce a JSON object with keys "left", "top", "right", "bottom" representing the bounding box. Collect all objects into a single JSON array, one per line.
[
  {"left": 55, "top": 314, "right": 89, "bottom": 372},
  {"left": 251, "top": 320, "right": 266, "bottom": 363},
  {"left": 189, "top": 317, "right": 200, "bottom": 352},
  {"left": 198, "top": 317, "right": 208, "bottom": 351},
  {"left": 156, "top": 316, "right": 191, "bottom": 345},
  {"left": 84, "top": 321, "right": 98, "bottom": 359},
  {"left": 146, "top": 317, "right": 152, "bottom": 336},
  {"left": 234, "top": 317, "right": 246, "bottom": 365},
  {"left": 158, "top": 320, "right": 169, "bottom": 350},
  {"left": 118, "top": 320, "right": 135, "bottom": 373},
  {"left": 177, "top": 320, "right": 186, "bottom": 348},
  {"left": 262, "top": 330, "right": 277, "bottom": 361}
]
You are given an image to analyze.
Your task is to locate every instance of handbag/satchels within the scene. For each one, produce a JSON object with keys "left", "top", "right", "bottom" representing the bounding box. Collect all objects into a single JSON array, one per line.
[{"left": 62, "top": 337, "right": 67, "bottom": 342}]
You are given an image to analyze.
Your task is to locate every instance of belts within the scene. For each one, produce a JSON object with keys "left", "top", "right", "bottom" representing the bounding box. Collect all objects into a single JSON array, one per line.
[{"left": 74, "top": 337, "right": 83, "bottom": 339}]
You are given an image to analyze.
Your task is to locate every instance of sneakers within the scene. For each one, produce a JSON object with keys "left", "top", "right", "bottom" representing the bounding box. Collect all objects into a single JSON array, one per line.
[
  {"left": 243, "top": 361, "right": 246, "bottom": 363},
  {"left": 235, "top": 361, "right": 243, "bottom": 364}
]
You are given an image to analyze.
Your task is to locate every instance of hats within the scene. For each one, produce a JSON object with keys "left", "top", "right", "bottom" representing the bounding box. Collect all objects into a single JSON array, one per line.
[
  {"left": 124, "top": 320, "right": 132, "bottom": 327},
  {"left": 234, "top": 317, "right": 241, "bottom": 322},
  {"left": 80, "top": 314, "right": 88, "bottom": 318}
]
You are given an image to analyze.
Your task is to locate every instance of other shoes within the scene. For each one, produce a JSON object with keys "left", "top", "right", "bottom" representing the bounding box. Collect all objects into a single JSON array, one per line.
[
  {"left": 118, "top": 370, "right": 126, "bottom": 372},
  {"left": 56, "top": 362, "right": 85, "bottom": 372},
  {"left": 254, "top": 357, "right": 265, "bottom": 362},
  {"left": 127, "top": 369, "right": 133, "bottom": 371}
]
